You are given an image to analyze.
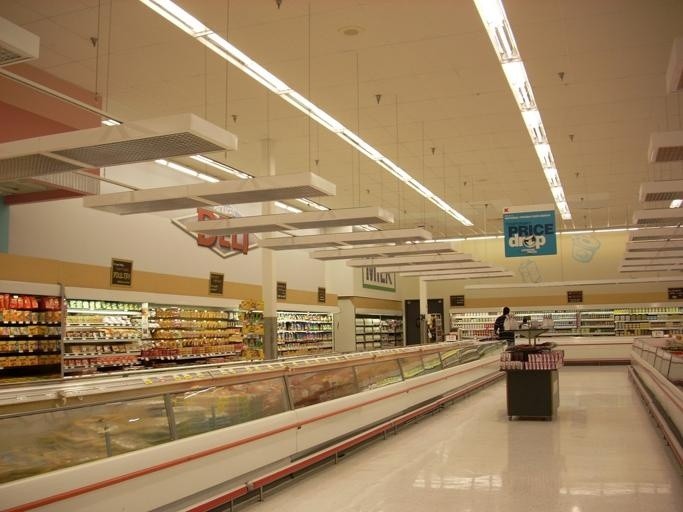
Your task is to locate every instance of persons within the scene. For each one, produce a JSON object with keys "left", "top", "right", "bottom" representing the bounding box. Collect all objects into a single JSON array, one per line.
[
  {"left": 519, "top": 318, "right": 530, "bottom": 338},
  {"left": 494, "top": 307, "right": 515, "bottom": 347}
]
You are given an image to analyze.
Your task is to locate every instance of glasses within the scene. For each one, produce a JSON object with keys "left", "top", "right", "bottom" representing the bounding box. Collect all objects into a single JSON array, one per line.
[{"left": 616, "top": 35, "right": 683, "bottom": 276}]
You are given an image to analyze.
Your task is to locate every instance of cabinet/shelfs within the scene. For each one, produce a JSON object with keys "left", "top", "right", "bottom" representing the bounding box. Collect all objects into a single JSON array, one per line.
[{"left": 0, "top": 280, "right": 683, "bottom": 512}]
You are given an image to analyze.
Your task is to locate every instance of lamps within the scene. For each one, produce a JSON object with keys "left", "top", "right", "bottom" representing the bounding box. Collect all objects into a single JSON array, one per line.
[
  {"left": 621, "top": 228, "right": 683, "bottom": 276},
  {"left": 648, "top": 130, "right": 683, "bottom": 161},
  {"left": 83, "top": 172, "right": 335, "bottom": 215},
  {"left": 263, "top": 228, "right": 431, "bottom": 250},
  {"left": 639, "top": 180, "right": 683, "bottom": 202},
  {"left": 188, "top": 206, "right": 395, "bottom": 235},
  {"left": 665, "top": 36, "right": 683, "bottom": 91},
  {"left": 142, "top": 2, "right": 474, "bottom": 225},
  {"left": 2, "top": 113, "right": 238, "bottom": 180},
  {"left": 310, "top": 242, "right": 512, "bottom": 282},
  {"left": 632, "top": 208, "right": 683, "bottom": 228}
]
